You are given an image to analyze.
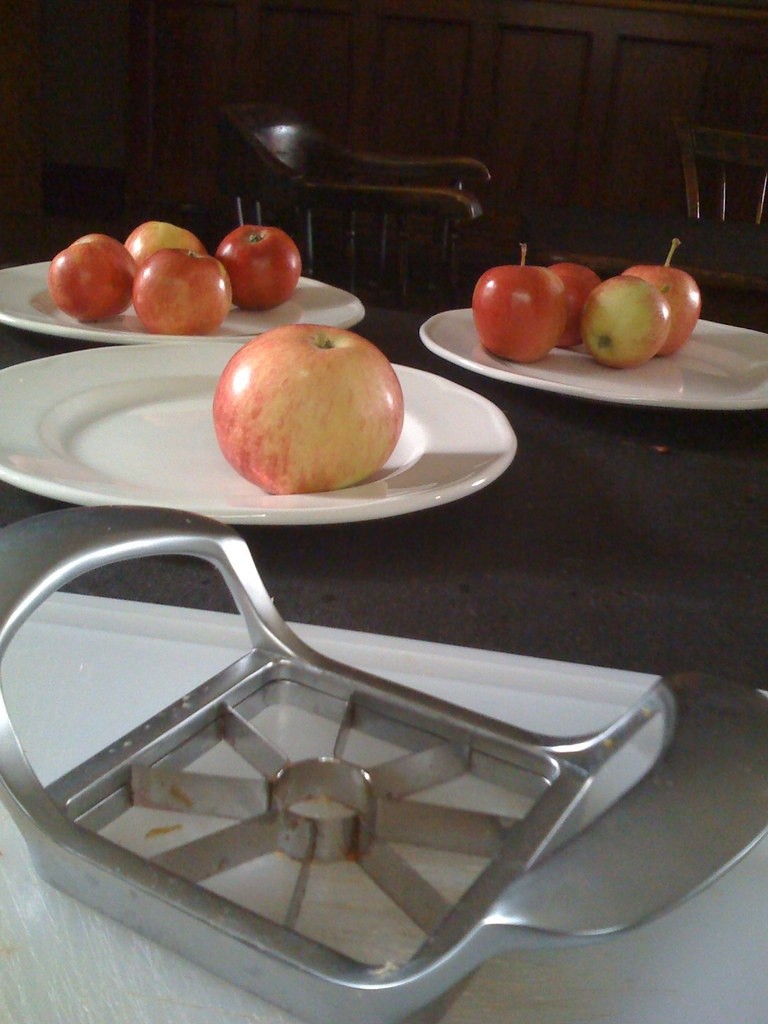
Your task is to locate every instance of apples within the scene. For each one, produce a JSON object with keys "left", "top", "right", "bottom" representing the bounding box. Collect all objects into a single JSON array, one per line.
[
  {"left": 620, "top": 238, "right": 701, "bottom": 357},
  {"left": 132, "top": 247, "right": 232, "bottom": 336},
  {"left": 213, "top": 323, "right": 403, "bottom": 495},
  {"left": 541, "top": 262, "right": 603, "bottom": 348},
  {"left": 124, "top": 221, "right": 208, "bottom": 268},
  {"left": 215, "top": 225, "right": 302, "bottom": 310},
  {"left": 48, "top": 234, "right": 139, "bottom": 323},
  {"left": 580, "top": 274, "right": 674, "bottom": 369},
  {"left": 474, "top": 237, "right": 567, "bottom": 363}
]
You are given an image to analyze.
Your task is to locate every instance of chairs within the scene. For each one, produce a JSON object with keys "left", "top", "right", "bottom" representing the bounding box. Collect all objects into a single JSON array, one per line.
[
  {"left": 671, "top": 118, "right": 768, "bottom": 228},
  {"left": 215, "top": 100, "right": 494, "bottom": 304}
]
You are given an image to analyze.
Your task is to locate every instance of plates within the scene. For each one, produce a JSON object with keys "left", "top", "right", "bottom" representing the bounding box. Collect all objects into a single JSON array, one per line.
[
  {"left": 418, "top": 304, "right": 767, "bottom": 413},
  {"left": 0, "top": 257, "right": 367, "bottom": 350}
]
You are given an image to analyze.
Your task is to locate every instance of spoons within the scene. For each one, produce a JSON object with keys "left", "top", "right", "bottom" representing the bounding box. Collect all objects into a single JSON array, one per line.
[{"left": 1, "top": 342, "right": 516, "bottom": 524}]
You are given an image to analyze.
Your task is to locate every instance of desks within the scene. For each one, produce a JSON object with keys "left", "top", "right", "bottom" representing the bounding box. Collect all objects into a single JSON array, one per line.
[
  {"left": 519, "top": 208, "right": 768, "bottom": 333},
  {"left": 0, "top": 259, "right": 768, "bottom": 1024}
]
